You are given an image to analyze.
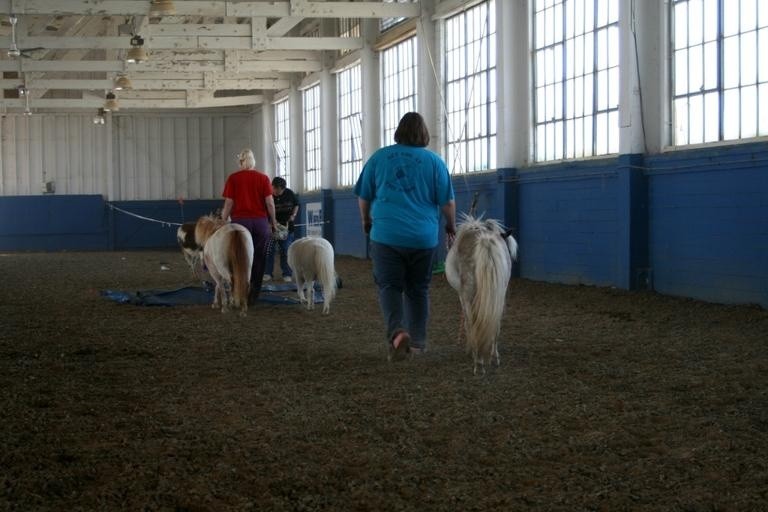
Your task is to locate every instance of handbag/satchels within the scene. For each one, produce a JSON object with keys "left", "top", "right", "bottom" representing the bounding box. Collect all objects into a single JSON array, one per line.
[{"left": 269, "top": 221, "right": 288, "bottom": 240}]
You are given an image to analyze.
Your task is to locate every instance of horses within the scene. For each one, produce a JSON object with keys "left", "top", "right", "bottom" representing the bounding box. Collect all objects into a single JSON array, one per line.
[
  {"left": 444, "top": 209, "right": 520, "bottom": 376},
  {"left": 286, "top": 236, "right": 339, "bottom": 315},
  {"left": 194, "top": 210, "right": 255, "bottom": 317},
  {"left": 176, "top": 222, "right": 202, "bottom": 282}
]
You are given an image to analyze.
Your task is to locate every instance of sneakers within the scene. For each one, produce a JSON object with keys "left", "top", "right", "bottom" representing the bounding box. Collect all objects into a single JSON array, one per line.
[
  {"left": 388, "top": 332, "right": 411, "bottom": 362},
  {"left": 262, "top": 274, "right": 272, "bottom": 281},
  {"left": 283, "top": 275, "right": 292, "bottom": 281}
]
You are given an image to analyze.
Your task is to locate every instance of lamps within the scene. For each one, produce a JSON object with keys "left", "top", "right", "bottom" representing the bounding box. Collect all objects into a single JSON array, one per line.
[
  {"left": 92, "top": 106, "right": 105, "bottom": 127},
  {"left": 111, "top": 71, "right": 131, "bottom": 91},
  {"left": 103, "top": 92, "right": 119, "bottom": 113},
  {"left": 123, "top": 14, "right": 148, "bottom": 64}
]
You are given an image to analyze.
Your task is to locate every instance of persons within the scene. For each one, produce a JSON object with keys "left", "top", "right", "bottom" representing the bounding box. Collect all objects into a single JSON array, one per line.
[
  {"left": 262, "top": 176, "right": 301, "bottom": 284},
  {"left": 218, "top": 150, "right": 281, "bottom": 305},
  {"left": 350, "top": 112, "right": 459, "bottom": 361}
]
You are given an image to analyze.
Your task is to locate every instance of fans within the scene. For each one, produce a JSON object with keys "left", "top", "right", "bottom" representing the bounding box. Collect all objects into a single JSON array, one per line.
[
  {"left": 0, "top": 14, "right": 46, "bottom": 60},
  {"left": 1, "top": 70, "right": 44, "bottom": 119}
]
